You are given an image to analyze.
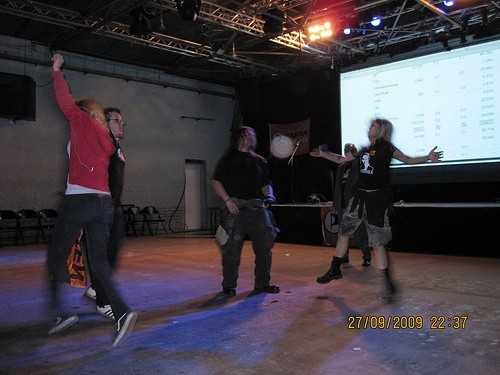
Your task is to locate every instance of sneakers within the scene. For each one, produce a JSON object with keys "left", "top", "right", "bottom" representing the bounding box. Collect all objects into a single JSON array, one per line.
[
  {"left": 110, "top": 311, "right": 138, "bottom": 347},
  {"left": 96, "top": 304, "right": 116, "bottom": 320},
  {"left": 48, "top": 312, "right": 79, "bottom": 334},
  {"left": 85, "top": 286, "right": 97, "bottom": 300}
]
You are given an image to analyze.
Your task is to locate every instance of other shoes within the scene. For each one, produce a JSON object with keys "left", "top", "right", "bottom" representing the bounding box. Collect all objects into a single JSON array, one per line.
[
  {"left": 254, "top": 284, "right": 280, "bottom": 293},
  {"left": 341, "top": 254, "right": 349, "bottom": 263},
  {"left": 362, "top": 255, "right": 372, "bottom": 266},
  {"left": 220, "top": 282, "right": 236, "bottom": 296}
]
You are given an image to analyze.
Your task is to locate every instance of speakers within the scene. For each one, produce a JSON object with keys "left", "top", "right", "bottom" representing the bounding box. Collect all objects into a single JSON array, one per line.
[{"left": 0, "top": 72, "right": 37, "bottom": 122}]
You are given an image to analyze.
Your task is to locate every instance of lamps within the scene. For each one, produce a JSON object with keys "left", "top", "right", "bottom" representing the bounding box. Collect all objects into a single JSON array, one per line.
[
  {"left": 260, "top": 9, "right": 285, "bottom": 33},
  {"left": 129, "top": 13, "right": 153, "bottom": 36},
  {"left": 177, "top": 0, "right": 201, "bottom": 22}
]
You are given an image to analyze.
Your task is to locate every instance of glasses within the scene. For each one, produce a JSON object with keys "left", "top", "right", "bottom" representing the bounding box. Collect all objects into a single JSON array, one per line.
[{"left": 110, "top": 119, "right": 125, "bottom": 125}]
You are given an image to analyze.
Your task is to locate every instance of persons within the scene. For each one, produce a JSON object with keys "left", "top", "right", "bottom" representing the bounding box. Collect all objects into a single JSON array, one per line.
[
  {"left": 309, "top": 119, "right": 444, "bottom": 306},
  {"left": 85, "top": 107, "right": 127, "bottom": 322},
  {"left": 209, "top": 127, "right": 280, "bottom": 297},
  {"left": 46, "top": 53, "right": 138, "bottom": 350}
]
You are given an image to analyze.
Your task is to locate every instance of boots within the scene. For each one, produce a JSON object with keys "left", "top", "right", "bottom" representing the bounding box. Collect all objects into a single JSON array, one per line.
[
  {"left": 379, "top": 267, "right": 396, "bottom": 294},
  {"left": 316, "top": 260, "right": 343, "bottom": 284}
]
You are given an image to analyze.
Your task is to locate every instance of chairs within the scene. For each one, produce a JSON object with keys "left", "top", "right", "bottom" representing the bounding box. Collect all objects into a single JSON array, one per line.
[
  {"left": 121, "top": 205, "right": 168, "bottom": 237},
  {"left": 17, "top": 209, "right": 46, "bottom": 246},
  {"left": 38, "top": 209, "right": 58, "bottom": 243},
  {"left": 0, "top": 209, "right": 21, "bottom": 246}
]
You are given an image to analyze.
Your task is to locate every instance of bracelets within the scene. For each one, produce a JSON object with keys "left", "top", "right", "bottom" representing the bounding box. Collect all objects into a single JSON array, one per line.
[{"left": 224, "top": 198, "right": 233, "bottom": 205}]
